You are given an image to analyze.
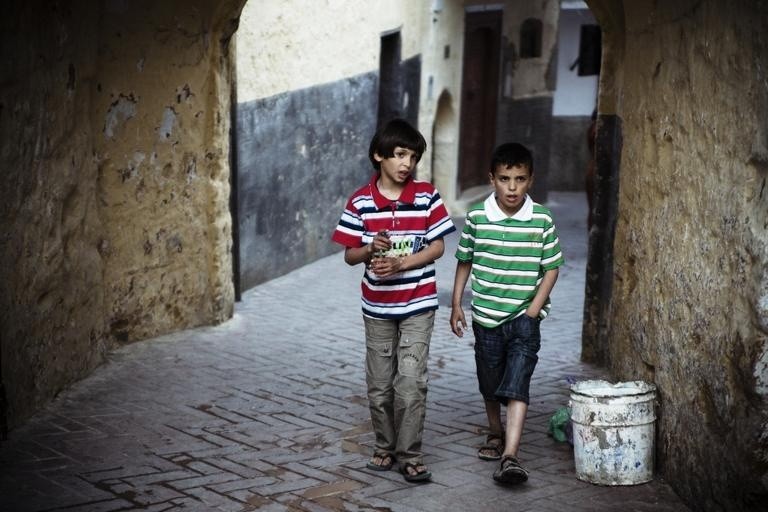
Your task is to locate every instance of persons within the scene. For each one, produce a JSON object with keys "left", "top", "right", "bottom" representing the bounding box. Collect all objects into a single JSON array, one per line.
[
  {"left": 450, "top": 144, "right": 565, "bottom": 486},
  {"left": 333, "top": 119, "right": 457, "bottom": 481}
]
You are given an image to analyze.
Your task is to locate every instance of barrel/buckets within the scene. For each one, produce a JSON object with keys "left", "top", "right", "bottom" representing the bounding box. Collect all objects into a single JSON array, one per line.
[{"left": 565, "top": 378, "right": 658, "bottom": 488}]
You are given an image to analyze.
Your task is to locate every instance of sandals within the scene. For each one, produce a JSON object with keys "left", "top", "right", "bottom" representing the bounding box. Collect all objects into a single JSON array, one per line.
[
  {"left": 478, "top": 430, "right": 505, "bottom": 461},
  {"left": 493, "top": 455, "right": 528, "bottom": 485}
]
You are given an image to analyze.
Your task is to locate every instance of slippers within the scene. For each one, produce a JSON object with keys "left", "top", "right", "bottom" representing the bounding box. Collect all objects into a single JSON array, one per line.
[
  {"left": 368, "top": 452, "right": 393, "bottom": 471},
  {"left": 399, "top": 463, "right": 431, "bottom": 481}
]
